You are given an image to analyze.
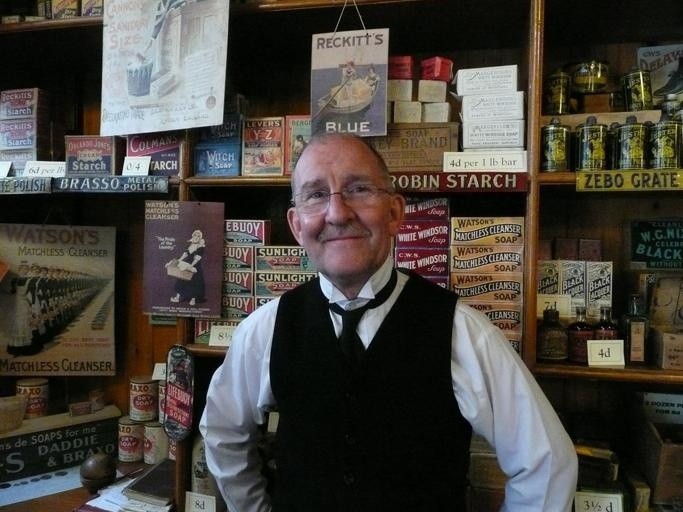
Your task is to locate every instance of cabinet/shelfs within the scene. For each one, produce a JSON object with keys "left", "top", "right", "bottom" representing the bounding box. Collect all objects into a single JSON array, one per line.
[{"left": 0, "top": 0, "right": 682, "bottom": 512}]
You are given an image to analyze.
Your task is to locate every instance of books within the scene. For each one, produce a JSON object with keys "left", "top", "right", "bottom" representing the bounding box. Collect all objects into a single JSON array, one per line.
[{"left": 120, "top": 457, "right": 176, "bottom": 506}]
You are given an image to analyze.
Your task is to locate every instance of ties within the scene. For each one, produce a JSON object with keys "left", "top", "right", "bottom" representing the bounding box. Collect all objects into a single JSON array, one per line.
[{"left": 331, "top": 269, "right": 397, "bottom": 352}]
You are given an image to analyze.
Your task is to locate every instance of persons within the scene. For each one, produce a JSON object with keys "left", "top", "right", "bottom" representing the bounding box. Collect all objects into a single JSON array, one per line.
[
  {"left": 363, "top": 62, "right": 381, "bottom": 95},
  {"left": 196, "top": 132, "right": 581, "bottom": 511},
  {"left": 342, "top": 61, "right": 357, "bottom": 104}
]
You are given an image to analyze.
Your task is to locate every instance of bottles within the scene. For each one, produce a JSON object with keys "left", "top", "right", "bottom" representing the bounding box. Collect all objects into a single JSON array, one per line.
[
  {"left": 546, "top": 63, "right": 676, "bottom": 173},
  {"left": 542, "top": 298, "right": 651, "bottom": 368}
]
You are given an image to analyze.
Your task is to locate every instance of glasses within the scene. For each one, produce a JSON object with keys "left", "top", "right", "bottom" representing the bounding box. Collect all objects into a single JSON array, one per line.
[{"left": 291, "top": 185, "right": 393, "bottom": 216}]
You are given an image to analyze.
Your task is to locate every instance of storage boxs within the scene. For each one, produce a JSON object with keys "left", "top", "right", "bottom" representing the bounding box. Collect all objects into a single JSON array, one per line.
[
  {"left": 358, "top": 64, "right": 526, "bottom": 173},
  {"left": 632, "top": 405, "right": 682, "bottom": 512}
]
